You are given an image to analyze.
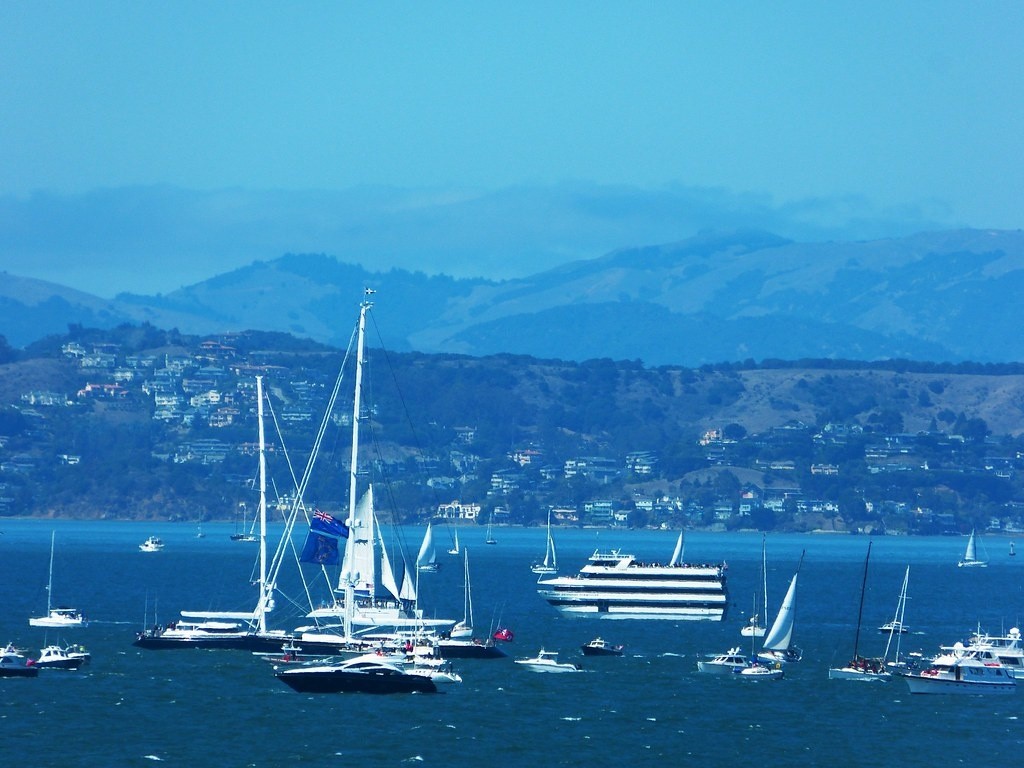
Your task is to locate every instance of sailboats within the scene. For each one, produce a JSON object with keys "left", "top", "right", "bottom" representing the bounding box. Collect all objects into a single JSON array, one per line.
[
  {"left": 27, "top": 528, "right": 83, "bottom": 628},
  {"left": 447, "top": 521, "right": 459, "bottom": 554},
  {"left": 825, "top": 547, "right": 903, "bottom": 683},
  {"left": 696, "top": 538, "right": 804, "bottom": 681},
  {"left": 484, "top": 511, "right": 498, "bottom": 544},
  {"left": 135, "top": 300, "right": 503, "bottom": 692},
  {"left": 412, "top": 523, "right": 439, "bottom": 574}
]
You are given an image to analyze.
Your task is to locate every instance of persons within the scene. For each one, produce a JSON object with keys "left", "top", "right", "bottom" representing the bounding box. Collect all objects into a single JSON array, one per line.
[
  {"left": 440, "top": 629, "right": 452, "bottom": 639},
  {"left": 283, "top": 652, "right": 299, "bottom": 661},
  {"left": 345, "top": 641, "right": 414, "bottom": 655},
  {"left": 847, "top": 658, "right": 886, "bottom": 674},
  {"left": 920, "top": 666, "right": 938, "bottom": 676},
  {"left": 151, "top": 621, "right": 176, "bottom": 637},
  {"left": 595, "top": 560, "right": 724, "bottom": 568}
]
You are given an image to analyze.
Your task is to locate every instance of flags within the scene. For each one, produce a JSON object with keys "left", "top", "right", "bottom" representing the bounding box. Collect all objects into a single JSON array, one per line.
[{"left": 298, "top": 507, "right": 351, "bottom": 565}]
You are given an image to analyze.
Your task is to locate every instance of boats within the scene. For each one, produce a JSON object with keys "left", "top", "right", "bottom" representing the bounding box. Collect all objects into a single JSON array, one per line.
[
  {"left": 515, "top": 647, "right": 580, "bottom": 675},
  {"left": 897, "top": 564, "right": 1024, "bottom": 694},
  {"left": 534, "top": 533, "right": 727, "bottom": 622},
  {"left": 136, "top": 536, "right": 164, "bottom": 553},
  {"left": 583, "top": 638, "right": 624, "bottom": 657},
  {"left": 0, "top": 631, "right": 90, "bottom": 677}
]
